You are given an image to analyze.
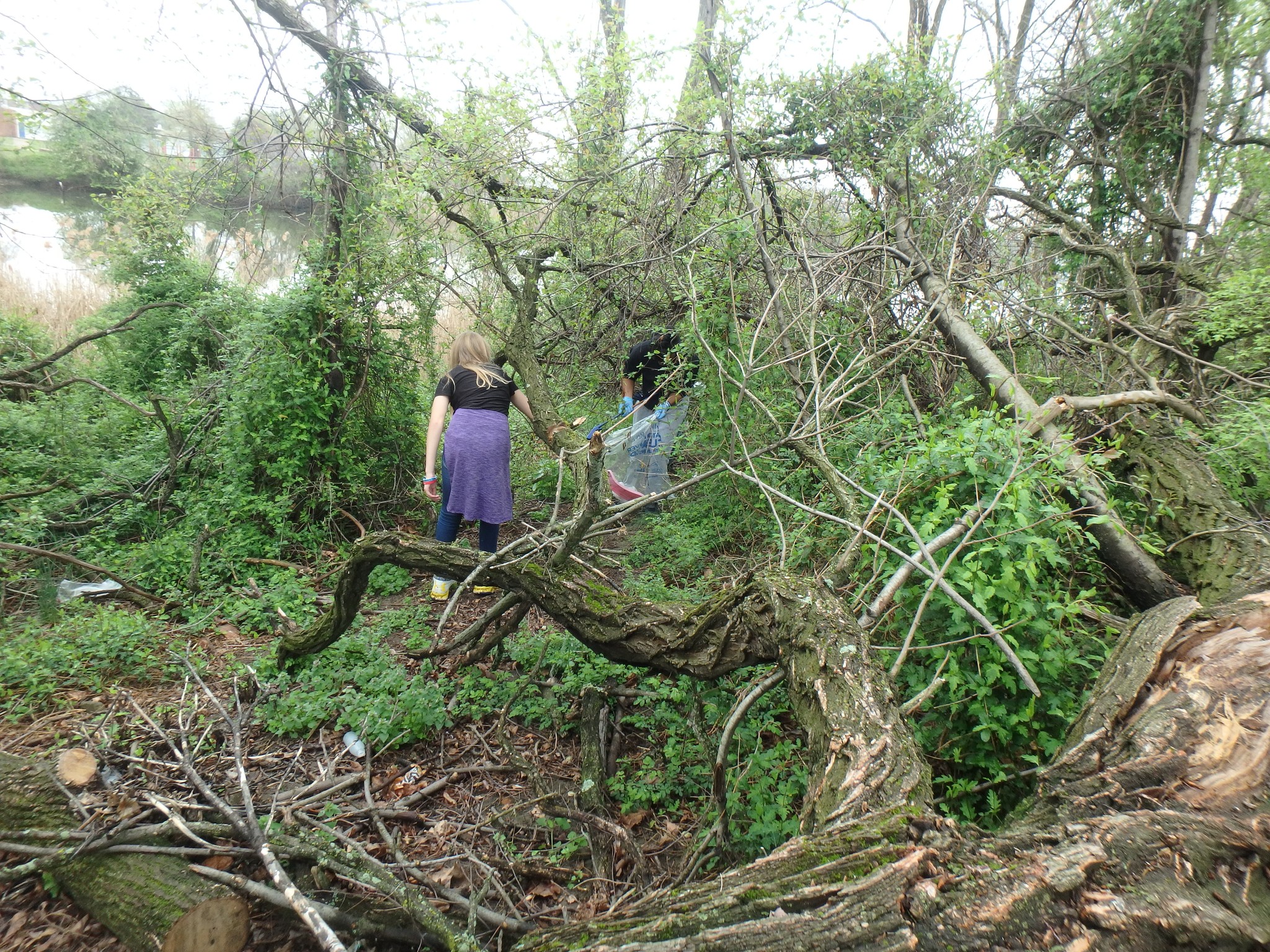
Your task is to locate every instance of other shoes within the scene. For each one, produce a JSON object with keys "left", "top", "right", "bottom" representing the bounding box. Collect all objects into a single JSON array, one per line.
[{"left": 644, "top": 504, "right": 661, "bottom": 516}]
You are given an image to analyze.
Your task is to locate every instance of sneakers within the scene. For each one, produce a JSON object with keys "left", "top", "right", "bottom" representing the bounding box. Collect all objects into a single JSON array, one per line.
[
  {"left": 472, "top": 584, "right": 503, "bottom": 595},
  {"left": 430, "top": 577, "right": 458, "bottom": 601}
]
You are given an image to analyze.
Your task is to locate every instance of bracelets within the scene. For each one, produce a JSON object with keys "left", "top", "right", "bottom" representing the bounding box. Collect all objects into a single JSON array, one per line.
[
  {"left": 423, "top": 479, "right": 437, "bottom": 484},
  {"left": 423, "top": 475, "right": 437, "bottom": 482}
]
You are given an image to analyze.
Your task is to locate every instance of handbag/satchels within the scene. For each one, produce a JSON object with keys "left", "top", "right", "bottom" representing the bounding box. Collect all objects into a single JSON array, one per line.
[{"left": 603, "top": 416, "right": 674, "bottom": 504}]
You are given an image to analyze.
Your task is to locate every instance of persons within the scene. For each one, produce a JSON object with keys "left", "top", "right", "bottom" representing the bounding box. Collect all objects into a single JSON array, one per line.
[
  {"left": 423, "top": 330, "right": 533, "bottom": 600},
  {"left": 618, "top": 325, "right": 699, "bottom": 497}
]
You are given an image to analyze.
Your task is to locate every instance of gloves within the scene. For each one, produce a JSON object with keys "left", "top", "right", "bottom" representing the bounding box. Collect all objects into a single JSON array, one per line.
[
  {"left": 618, "top": 397, "right": 634, "bottom": 417},
  {"left": 655, "top": 401, "right": 670, "bottom": 419}
]
